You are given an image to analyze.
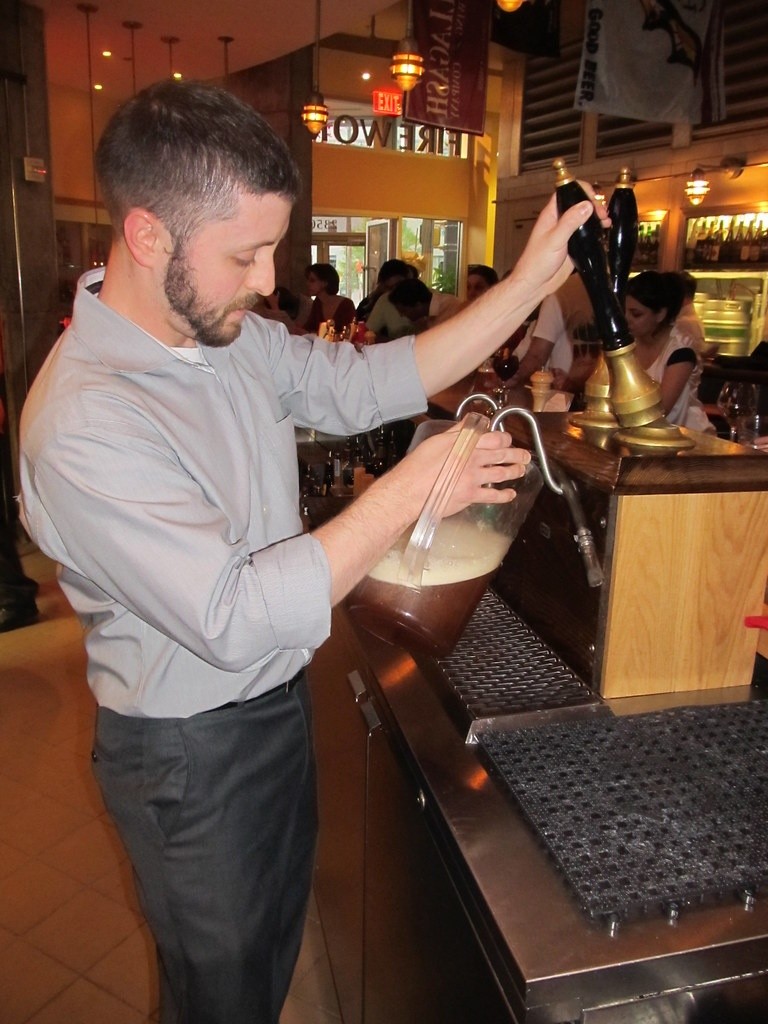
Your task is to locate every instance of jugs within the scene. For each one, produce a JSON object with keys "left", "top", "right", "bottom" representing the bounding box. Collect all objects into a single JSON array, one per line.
[{"left": 349, "top": 420, "right": 546, "bottom": 661}]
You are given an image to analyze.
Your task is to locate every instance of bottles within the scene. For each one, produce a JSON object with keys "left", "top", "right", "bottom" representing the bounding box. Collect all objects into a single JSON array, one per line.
[
  {"left": 638, "top": 224, "right": 660, "bottom": 264},
  {"left": 472, "top": 357, "right": 495, "bottom": 392},
  {"left": 301, "top": 424, "right": 388, "bottom": 497},
  {"left": 686, "top": 218, "right": 768, "bottom": 263}
]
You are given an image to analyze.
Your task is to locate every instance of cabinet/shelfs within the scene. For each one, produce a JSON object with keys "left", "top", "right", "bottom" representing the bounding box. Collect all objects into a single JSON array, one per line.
[{"left": 306, "top": 383, "right": 767, "bottom": 1024}]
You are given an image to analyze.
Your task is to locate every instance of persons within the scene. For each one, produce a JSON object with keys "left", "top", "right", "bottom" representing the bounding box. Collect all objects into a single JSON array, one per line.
[
  {"left": 356, "top": 260, "right": 410, "bottom": 323},
  {"left": 388, "top": 279, "right": 468, "bottom": 328},
  {"left": 505, "top": 272, "right": 595, "bottom": 388},
  {"left": 625, "top": 270, "right": 717, "bottom": 436},
  {"left": 16, "top": 80, "right": 613, "bottom": 1024},
  {"left": 664, "top": 270, "right": 704, "bottom": 351},
  {"left": 407, "top": 263, "right": 418, "bottom": 278},
  {"left": 467, "top": 264, "right": 498, "bottom": 303},
  {"left": 266, "top": 263, "right": 355, "bottom": 334}
]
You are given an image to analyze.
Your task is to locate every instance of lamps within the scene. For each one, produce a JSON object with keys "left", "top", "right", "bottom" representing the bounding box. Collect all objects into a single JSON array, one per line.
[
  {"left": 684, "top": 167, "right": 710, "bottom": 207},
  {"left": 301, "top": 0, "right": 328, "bottom": 136},
  {"left": 388, "top": 1, "right": 425, "bottom": 92}
]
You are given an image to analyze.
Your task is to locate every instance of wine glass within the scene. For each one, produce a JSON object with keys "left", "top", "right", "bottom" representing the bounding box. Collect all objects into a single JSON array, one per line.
[
  {"left": 717, "top": 382, "right": 759, "bottom": 442},
  {"left": 493, "top": 351, "right": 520, "bottom": 394}
]
